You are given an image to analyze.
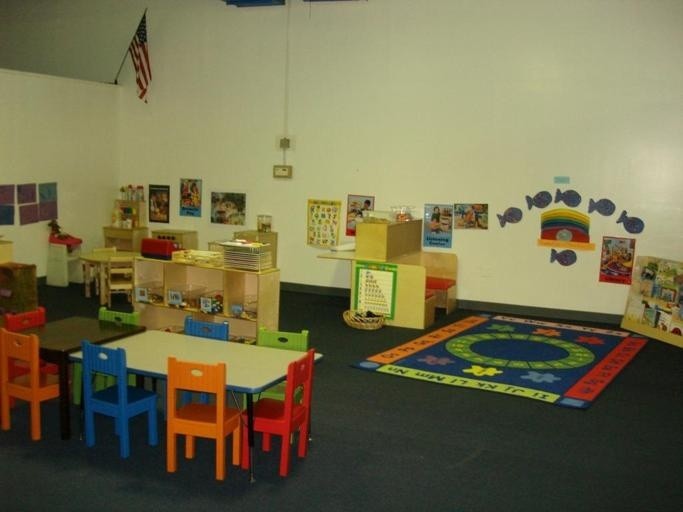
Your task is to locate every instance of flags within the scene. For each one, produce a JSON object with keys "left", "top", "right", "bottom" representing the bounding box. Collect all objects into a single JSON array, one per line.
[{"left": 129, "top": 15, "right": 152, "bottom": 104}]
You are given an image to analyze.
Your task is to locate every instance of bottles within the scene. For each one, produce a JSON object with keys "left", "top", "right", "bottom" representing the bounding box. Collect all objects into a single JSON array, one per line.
[{"left": 120, "top": 185, "right": 144, "bottom": 200}]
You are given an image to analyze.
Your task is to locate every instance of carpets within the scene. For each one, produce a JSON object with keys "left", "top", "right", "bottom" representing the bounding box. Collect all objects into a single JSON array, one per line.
[{"left": 350, "top": 313, "right": 649, "bottom": 409}]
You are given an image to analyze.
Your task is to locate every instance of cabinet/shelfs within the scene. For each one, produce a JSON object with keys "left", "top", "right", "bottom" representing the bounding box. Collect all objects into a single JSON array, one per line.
[
  {"left": 151, "top": 229, "right": 199, "bottom": 250},
  {"left": 235, "top": 230, "right": 278, "bottom": 266},
  {"left": 132, "top": 251, "right": 280, "bottom": 345},
  {"left": 103, "top": 199, "right": 149, "bottom": 252}
]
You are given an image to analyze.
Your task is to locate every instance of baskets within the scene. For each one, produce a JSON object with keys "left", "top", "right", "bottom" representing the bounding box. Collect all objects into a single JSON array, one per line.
[{"left": 342, "top": 310, "right": 385, "bottom": 330}]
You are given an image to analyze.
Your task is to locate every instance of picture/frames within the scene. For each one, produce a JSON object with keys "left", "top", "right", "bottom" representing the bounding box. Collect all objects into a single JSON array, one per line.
[{"left": 422, "top": 203, "right": 455, "bottom": 253}]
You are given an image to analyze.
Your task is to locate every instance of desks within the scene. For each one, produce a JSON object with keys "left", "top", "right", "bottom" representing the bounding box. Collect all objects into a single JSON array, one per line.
[
  {"left": 318, "top": 219, "right": 458, "bottom": 329},
  {"left": 15, "top": 316, "right": 148, "bottom": 441},
  {"left": 79, "top": 251, "right": 141, "bottom": 298},
  {"left": 69, "top": 328, "right": 324, "bottom": 482}
]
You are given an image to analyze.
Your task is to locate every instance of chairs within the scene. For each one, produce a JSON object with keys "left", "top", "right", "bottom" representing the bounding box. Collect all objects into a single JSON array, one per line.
[
  {"left": 81, "top": 337, "right": 160, "bottom": 459},
  {"left": 92, "top": 246, "right": 117, "bottom": 296},
  {"left": 179, "top": 314, "right": 231, "bottom": 405},
  {"left": 105, "top": 256, "right": 136, "bottom": 308},
  {"left": 1, "top": 325, "right": 59, "bottom": 442},
  {"left": 166, "top": 355, "right": 242, "bottom": 482},
  {"left": 68, "top": 307, "right": 140, "bottom": 408},
  {"left": 242, "top": 326, "right": 311, "bottom": 444},
  {"left": 2, "top": 308, "right": 58, "bottom": 406},
  {"left": 240, "top": 347, "right": 316, "bottom": 478}
]
